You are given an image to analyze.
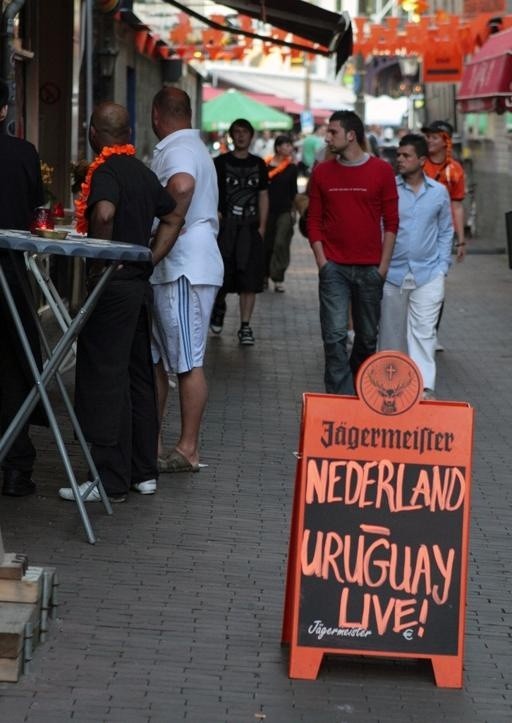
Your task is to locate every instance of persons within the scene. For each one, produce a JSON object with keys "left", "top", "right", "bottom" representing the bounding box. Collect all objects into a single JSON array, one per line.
[
  {"left": 1, "top": 82, "right": 43, "bottom": 498},
  {"left": 377, "top": 133, "right": 456, "bottom": 401},
  {"left": 207, "top": 124, "right": 332, "bottom": 167},
  {"left": 419, "top": 120, "right": 466, "bottom": 354},
  {"left": 56, "top": 99, "right": 185, "bottom": 504},
  {"left": 145, "top": 86, "right": 226, "bottom": 475},
  {"left": 366, "top": 123, "right": 421, "bottom": 175},
  {"left": 209, "top": 117, "right": 272, "bottom": 346},
  {"left": 306, "top": 110, "right": 401, "bottom": 397},
  {"left": 261, "top": 134, "right": 312, "bottom": 294}
]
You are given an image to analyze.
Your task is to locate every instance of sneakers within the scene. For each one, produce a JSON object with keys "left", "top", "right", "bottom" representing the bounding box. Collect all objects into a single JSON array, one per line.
[
  {"left": 433, "top": 344, "right": 445, "bottom": 352},
  {"left": 237, "top": 325, "right": 255, "bottom": 346},
  {"left": 132, "top": 477, "right": 159, "bottom": 496},
  {"left": 58, "top": 479, "right": 128, "bottom": 503},
  {"left": 2, "top": 470, "right": 40, "bottom": 497},
  {"left": 209, "top": 302, "right": 227, "bottom": 334},
  {"left": 347, "top": 330, "right": 356, "bottom": 346},
  {"left": 275, "top": 284, "right": 286, "bottom": 294}
]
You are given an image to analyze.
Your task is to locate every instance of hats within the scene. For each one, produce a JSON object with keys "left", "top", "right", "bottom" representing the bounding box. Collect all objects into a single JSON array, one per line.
[{"left": 420, "top": 119, "right": 453, "bottom": 137}]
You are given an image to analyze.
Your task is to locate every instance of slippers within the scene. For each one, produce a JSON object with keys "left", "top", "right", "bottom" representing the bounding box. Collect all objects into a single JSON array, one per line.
[{"left": 155, "top": 449, "right": 202, "bottom": 474}]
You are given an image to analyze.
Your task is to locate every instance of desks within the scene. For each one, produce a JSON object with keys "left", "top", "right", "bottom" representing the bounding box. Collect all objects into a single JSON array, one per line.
[{"left": 1, "top": 229, "right": 152, "bottom": 544}]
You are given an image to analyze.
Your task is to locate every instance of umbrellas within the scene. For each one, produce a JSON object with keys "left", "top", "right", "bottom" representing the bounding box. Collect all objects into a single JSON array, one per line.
[{"left": 201, "top": 86, "right": 293, "bottom": 133}]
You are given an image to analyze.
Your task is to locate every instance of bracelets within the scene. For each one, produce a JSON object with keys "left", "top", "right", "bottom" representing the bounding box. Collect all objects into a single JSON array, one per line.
[{"left": 455, "top": 241, "right": 466, "bottom": 248}]
